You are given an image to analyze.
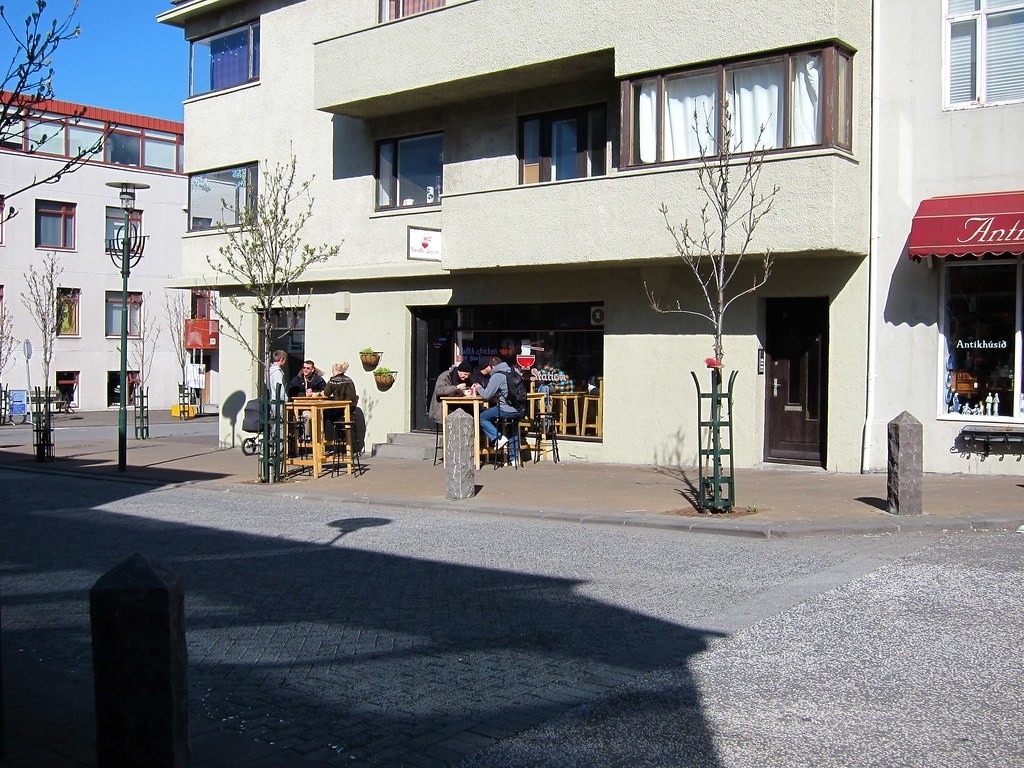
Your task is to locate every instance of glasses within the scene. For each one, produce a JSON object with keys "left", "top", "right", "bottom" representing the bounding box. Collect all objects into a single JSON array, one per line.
[{"left": 303, "top": 367, "right": 310, "bottom": 369}]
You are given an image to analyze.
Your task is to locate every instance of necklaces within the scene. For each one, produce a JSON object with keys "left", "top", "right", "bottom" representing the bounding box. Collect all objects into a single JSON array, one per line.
[{"left": 305, "top": 377, "right": 314, "bottom": 382}]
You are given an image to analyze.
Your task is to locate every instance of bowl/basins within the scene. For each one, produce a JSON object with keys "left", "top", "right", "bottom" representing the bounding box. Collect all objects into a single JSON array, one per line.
[
  {"left": 462, "top": 390, "right": 471, "bottom": 396},
  {"left": 310, "top": 392, "right": 319, "bottom": 397}
]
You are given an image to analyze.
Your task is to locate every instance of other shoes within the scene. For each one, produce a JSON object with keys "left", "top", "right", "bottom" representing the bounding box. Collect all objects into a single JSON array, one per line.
[
  {"left": 504, "top": 459, "right": 519, "bottom": 467},
  {"left": 302, "top": 435, "right": 310, "bottom": 442},
  {"left": 323, "top": 449, "right": 334, "bottom": 455},
  {"left": 493, "top": 435, "right": 508, "bottom": 451}
]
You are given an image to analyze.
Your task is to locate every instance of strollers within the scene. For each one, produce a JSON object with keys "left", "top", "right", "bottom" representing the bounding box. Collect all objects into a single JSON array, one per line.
[{"left": 241, "top": 398, "right": 271, "bottom": 456}]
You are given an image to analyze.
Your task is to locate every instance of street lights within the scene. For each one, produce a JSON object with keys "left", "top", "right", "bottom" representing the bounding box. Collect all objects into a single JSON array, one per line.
[{"left": 105, "top": 181, "right": 151, "bottom": 473}]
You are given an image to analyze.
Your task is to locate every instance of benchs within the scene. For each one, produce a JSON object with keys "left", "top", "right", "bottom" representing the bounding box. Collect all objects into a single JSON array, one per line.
[{"left": 26, "top": 390, "right": 69, "bottom": 415}]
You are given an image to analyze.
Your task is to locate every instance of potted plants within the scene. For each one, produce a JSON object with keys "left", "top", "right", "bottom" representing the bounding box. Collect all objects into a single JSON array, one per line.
[
  {"left": 360, "top": 347, "right": 380, "bottom": 371},
  {"left": 372, "top": 367, "right": 396, "bottom": 391}
]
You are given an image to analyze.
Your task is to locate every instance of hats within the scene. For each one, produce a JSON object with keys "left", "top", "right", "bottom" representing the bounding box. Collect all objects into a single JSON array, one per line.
[
  {"left": 457, "top": 360, "right": 473, "bottom": 372},
  {"left": 478, "top": 357, "right": 490, "bottom": 371}
]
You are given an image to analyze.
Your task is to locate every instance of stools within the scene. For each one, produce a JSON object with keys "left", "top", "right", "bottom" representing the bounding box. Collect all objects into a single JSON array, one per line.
[
  {"left": 281, "top": 412, "right": 362, "bottom": 478},
  {"left": 493, "top": 416, "right": 524, "bottom": 470},
  {"left": 533, "top": 411, "right": 560, "bottom": 463}
]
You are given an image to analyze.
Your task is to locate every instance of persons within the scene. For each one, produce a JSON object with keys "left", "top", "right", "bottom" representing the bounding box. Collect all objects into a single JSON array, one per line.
[
  {"left": 428, "top": 360, "right": 476, "bottom": 425},
  {"left": 285, "top": 360, "right": 326, "bottom": 440},
  {"left": 478, "top": 357, "right": 526, "bottom": 466},
  {"left": 269, "top": 350, "right": 290, "bottom": 452},
  {"left": 472, "top": 357, "right": 492, "bottom": 388},
  {"left": 321, "top": 361, "right": 356, "bottom": 439},
  {"left": 511, "top": 360, "right": 573, "bottom": 430}
]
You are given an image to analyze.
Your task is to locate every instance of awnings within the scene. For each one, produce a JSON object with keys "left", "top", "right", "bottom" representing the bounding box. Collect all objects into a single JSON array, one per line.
[{"left": 907, "top": 190, "right": 1024, "bottom": 264}]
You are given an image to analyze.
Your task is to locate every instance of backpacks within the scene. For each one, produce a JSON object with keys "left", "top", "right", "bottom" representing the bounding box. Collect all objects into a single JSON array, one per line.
[{"left": 495, "top": 368, "right": 527, "bottom": 411}]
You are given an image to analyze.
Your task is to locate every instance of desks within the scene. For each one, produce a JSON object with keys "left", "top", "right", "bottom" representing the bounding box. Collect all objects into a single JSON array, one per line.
[
  {"left": 282, "top": 401, "right": 352, "bottom": 479},
  {"left": 549, "top": 392, "right": 581, "bottom": 436},
  {"left": 291, "top": 396, "right": 330, "bottom": 457},
  {"left": 440, "top": 392, "right": 548, "bottom": 471}
]
